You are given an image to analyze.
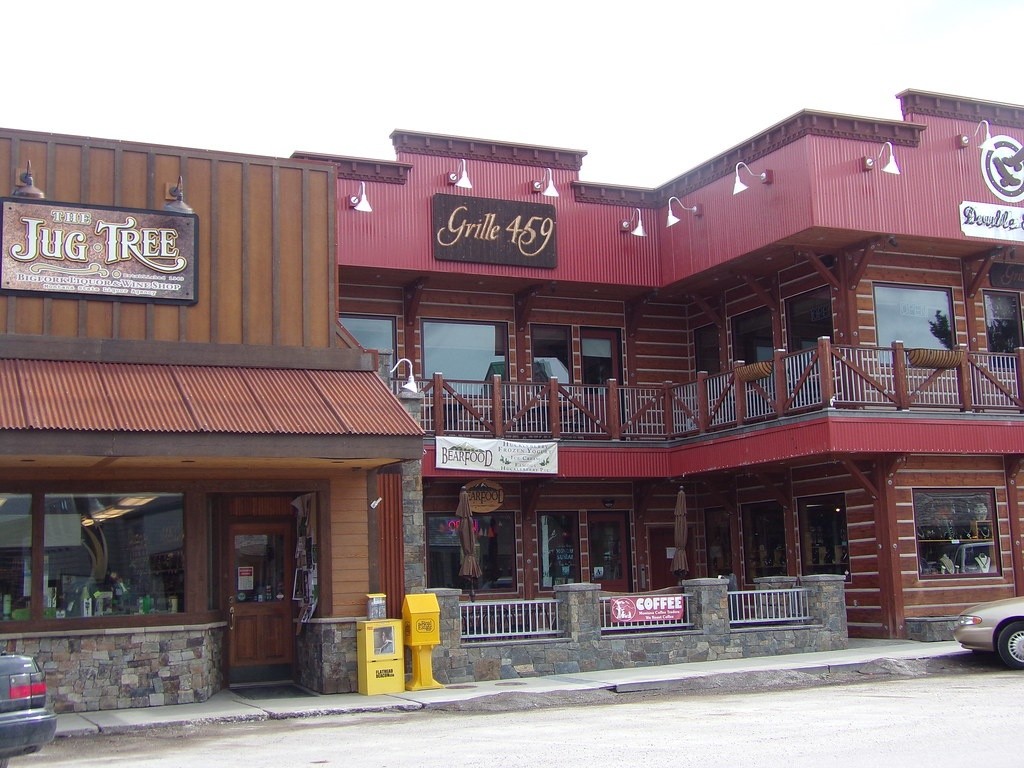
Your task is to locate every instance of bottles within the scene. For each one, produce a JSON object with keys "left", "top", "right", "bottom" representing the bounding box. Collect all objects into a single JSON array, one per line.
[
  {"left": 79, "top": 586, "right": 90, "bottom": 617},
  {"left": 975, "top": 503, "right": 987, "bottom": 520},
  {"left": 135, "top": 594, "right": 155, "bottom": 615},
  {"left": 254, "top": 584, "right": 272, "bottom": 602}
]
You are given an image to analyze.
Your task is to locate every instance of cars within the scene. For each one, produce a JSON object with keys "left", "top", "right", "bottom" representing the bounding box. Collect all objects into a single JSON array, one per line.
[
  {"left": 952, "top": 594, "right": 1024, "bottom": 671},
  {"left": 0, "top": 652, "right": 58, "bottom": 768},
  {"left": 953, "top": 540, "right": 996, "bottom": 574}
]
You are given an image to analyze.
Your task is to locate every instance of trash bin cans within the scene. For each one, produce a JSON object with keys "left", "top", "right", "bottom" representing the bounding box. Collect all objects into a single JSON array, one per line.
[
  {"left": 356, "top": 593, "right": 407, "bottom": 696},
  {"left": 400, "top": 592, "right": 441, "bottom": 692}
]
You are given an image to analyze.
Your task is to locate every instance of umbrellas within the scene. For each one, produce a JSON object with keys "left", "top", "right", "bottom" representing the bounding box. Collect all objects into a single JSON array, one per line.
[
  {"left": 671, "top": 486, "right": 690, "bottom": 582},
  {"left": 454, "top": 486, "right": 484, "bottom": 602}
]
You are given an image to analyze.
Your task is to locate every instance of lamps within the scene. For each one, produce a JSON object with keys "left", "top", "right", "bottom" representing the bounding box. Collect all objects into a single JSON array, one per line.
[
  {"left": 162, "top": 175, "right": 194, "bottom": 214},
  {"left": 861, "top": 141, "right": 900, "bottom": 175},
  {"left": 447, "top": 159, "right": 472, "bottom": 189},
  {"left": 620, "top": 207, "right": 647, "bottom": 237},
  {"left": 665, "top": 195, "right": 704, "bottom": 228},
  {"left": 348, "top": 181, "right": 373, "bottom": 212},
  {"left": 13, "top": 158, "right": 45, "bottom": 199},
  {"left": 390, "top": 358, "right": 418, "bottom": 394},
  {"left": 732, "top": 161, "right": 773, "bottom": 195},
  {"left": 531, "top": 167, "right": 559, "bottom": 196},
  {"left": 957, "top": 120, "right": 996, "bottom": 152}
]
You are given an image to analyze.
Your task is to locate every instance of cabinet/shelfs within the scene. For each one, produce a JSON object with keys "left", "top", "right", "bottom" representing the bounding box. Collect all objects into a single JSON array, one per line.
[
  {"left": 113, "top": 547, "right": 184, "bottom": 613},
  {"left": 746, "top": 519, "right": 997, "bottom": 580}
]
